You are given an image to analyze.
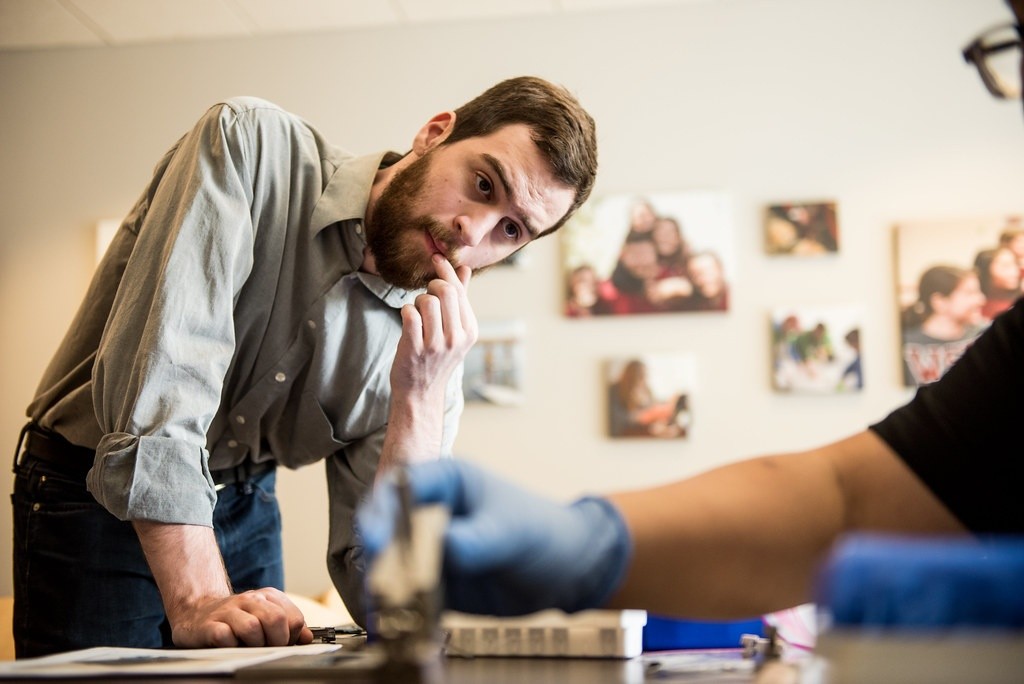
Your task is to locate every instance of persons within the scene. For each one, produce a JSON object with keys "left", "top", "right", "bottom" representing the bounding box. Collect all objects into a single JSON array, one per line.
[
  {"left": 893, "top": 222, "right": 1024, "bottom": 379},
  {"left": 561, "top": 201, "right": 728, "bottom": 313},
  {"left": 769, "top": 313, "right": 862, "bottom": 389},
  {"left": 761, "top": 199, "right": 839, "bottom": 249},
  {"left": 607, "top": 353, "right": 693, "bottom": 431},
  {"left": 10, "top": 76, "right": 597, "bottom": 653},
  {"left": 350, "top": 3, "right": 1024, "bottom": 636}
]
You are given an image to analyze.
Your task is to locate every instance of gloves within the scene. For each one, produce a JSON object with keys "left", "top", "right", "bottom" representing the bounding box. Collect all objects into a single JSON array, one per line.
[{"left": 356, "top": 455, "right": 631, "bottom": 618}]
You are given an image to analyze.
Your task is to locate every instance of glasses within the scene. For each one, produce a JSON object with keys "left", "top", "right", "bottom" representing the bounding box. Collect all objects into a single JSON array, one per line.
[{"left": 962, "top": 22, "right": 1024, "bottom": 100}]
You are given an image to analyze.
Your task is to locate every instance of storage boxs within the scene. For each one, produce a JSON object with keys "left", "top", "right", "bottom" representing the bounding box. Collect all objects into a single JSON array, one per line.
[{"left": 441, "top": 608, "right": 647, "bottom": 658}]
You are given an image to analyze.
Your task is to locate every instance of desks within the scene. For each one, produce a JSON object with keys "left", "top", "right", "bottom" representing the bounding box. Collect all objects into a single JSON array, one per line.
[{"left": 0, "top": 638, "right": 744, "bottom": 684}]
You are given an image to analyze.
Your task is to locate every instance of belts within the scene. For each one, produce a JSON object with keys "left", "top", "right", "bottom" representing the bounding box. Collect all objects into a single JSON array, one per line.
[{"left": 27, "top": 430, "right": 274, "bottom": 484}]
